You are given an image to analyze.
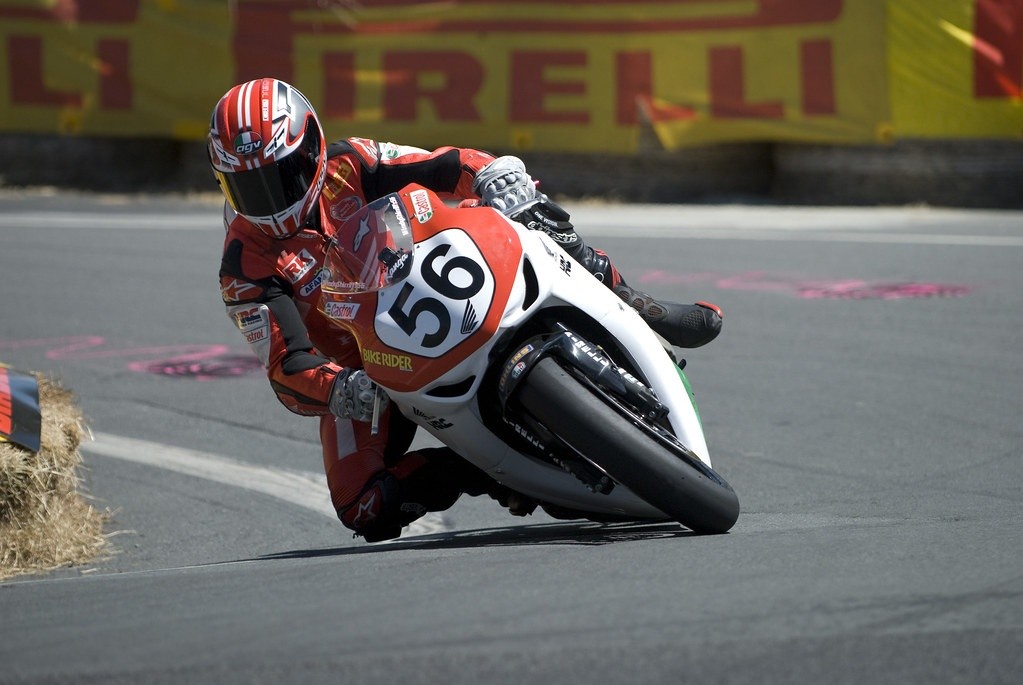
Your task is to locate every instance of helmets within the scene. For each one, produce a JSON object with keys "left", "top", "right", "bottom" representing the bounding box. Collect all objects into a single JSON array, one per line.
[{"left": 206, "top": 77, "right": 327, "bottom": 239}]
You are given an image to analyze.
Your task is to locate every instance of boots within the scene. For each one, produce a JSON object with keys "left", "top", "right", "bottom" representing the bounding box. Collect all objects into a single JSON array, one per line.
[
  {"left": 576, "top": 237, "right": 721, "bottom": 349},
  {"left": 419, "top": 438, "right": 529, "bottom": 514}
]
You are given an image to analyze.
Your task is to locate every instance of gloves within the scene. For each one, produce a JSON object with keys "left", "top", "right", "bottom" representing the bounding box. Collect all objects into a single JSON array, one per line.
[
  {"left": 472, "top": 153, "right": 541, "bottom": 220},
  {"left": 327, "top": 366, "right": 390, "bottom": 422}
]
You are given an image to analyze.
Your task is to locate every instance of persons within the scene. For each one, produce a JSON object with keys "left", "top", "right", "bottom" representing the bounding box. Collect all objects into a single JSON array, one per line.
[{"left": 206, "top": 77, "right": 724, "bottom": 544}]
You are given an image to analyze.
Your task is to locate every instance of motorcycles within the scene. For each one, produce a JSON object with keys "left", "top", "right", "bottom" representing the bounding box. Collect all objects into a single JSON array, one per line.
[{"left": 316, "top": 183, "right": 740, "bottom": 534}]
